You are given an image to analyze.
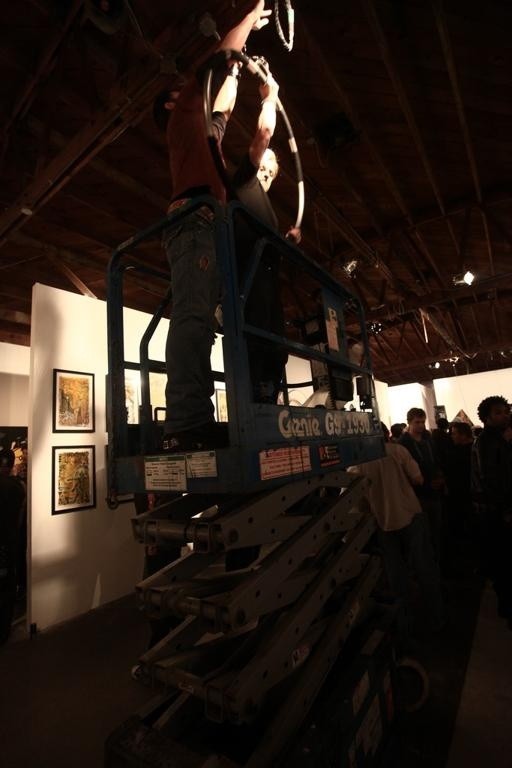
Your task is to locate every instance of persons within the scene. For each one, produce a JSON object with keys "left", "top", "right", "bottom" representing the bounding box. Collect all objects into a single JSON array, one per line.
[
  {"left": 344, "top": 394, "right": 511, "bottom": 633},
  {"left": 155, "top": 0, "right": 278, "bottom": 450},
  {"left": 1, "top": 447, "right": 28, "bottom": 622},
  {"left": 219, "top": 51, "right": 302, "bottom": 405}
]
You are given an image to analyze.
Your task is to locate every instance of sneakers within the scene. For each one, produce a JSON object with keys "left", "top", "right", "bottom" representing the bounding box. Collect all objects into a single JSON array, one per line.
[{"left": 167, "top": 423, "right": 231, "bottom": 452}]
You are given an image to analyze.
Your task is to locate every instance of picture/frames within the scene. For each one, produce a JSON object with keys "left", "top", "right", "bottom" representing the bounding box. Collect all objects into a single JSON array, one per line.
[
  {"left": 214, "top": 388, "right": 229, "bottom": 424},
  {"left": 52, "top": 368, "right": 141, "bottom": 515}
]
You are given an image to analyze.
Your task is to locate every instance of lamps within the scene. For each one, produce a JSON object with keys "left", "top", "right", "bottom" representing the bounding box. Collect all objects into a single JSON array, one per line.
[{"left": 454, "top": 270, "right": 475, "bottom": 288}]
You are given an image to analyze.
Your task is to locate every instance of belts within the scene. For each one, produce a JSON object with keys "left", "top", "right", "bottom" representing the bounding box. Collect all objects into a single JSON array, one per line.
[{"left": 166, "top": 197, "right": 215, "bottom": 225}]
